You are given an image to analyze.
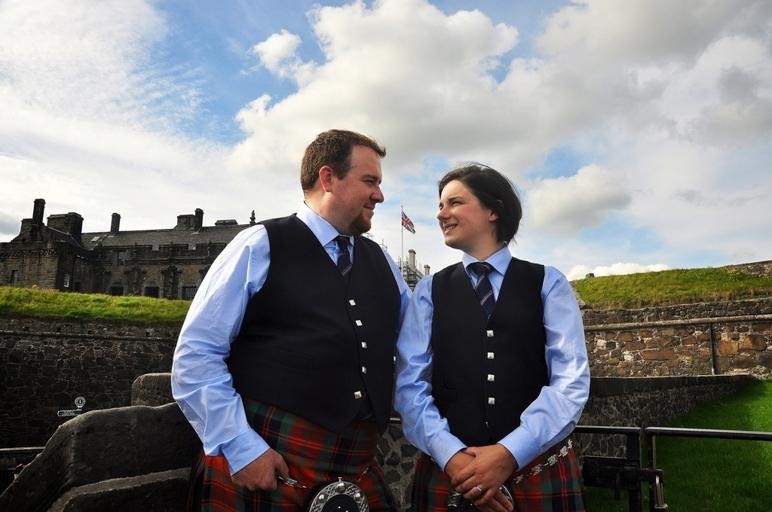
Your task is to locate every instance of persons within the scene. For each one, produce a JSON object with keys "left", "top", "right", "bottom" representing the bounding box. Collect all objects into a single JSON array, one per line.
[
  {"left": 394, "top": 166, "right": 591, "bottom": 512},
  {"left": 171, "top": 129, "right": 413, "bottom": 512}
]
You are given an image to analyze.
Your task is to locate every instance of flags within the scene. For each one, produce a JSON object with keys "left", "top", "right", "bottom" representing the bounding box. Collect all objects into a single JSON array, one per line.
[{"left": 402, "top": 212, "right": 416, "bottom": 235}]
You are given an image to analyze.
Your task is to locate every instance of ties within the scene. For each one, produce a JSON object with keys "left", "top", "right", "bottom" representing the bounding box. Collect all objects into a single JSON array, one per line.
[
  {"left": 471, "top": 262, "right": 494, "bottom": 315},
  {"left": 335, "top": 235, "right": 352, "bottom": 281}
]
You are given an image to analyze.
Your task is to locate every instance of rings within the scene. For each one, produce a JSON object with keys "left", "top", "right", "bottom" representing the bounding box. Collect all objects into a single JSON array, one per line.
[{"left": 476, "top": 486, "right": 483, "bottom": 491}]
